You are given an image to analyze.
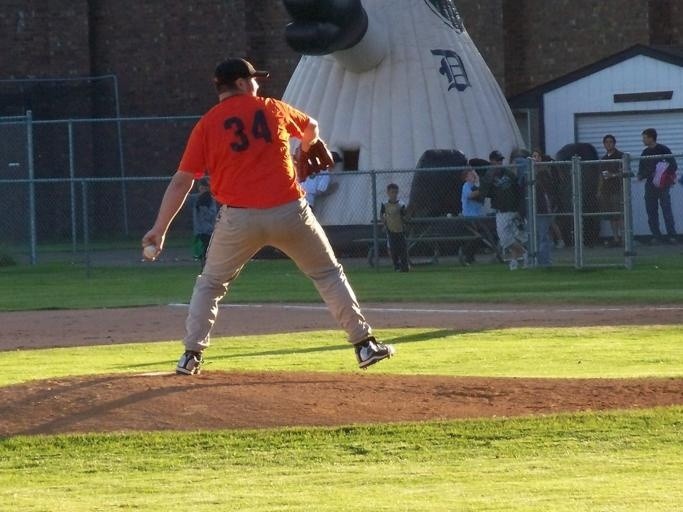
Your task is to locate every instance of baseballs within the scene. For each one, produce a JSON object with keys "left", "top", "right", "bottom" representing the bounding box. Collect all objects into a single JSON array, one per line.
[
  {"left": 143, "top": 245, "right": 157, "bottom": 259},
  {"left": 447, "top": 213, "right": 452, "bottom": 217}
]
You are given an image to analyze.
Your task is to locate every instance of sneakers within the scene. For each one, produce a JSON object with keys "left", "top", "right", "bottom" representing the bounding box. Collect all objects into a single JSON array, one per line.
[
  {"left": 463, "top": 240, "right": 566, "bottom": 271},
  {"left": 609, "top": 236, "right": 680, "bottom": 247},
  {"left": 355, "top": 335, "right": 395, "bottom": 370},
  {"left": 176, "top": 350, "right": 203, "bottom": 377},
  {"left": 393, "top": 261, "right": 409, "bottom": 273}
]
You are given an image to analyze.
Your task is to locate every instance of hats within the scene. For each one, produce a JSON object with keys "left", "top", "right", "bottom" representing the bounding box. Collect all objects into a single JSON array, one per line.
[
  {"left": 489, "top": 150, "right": 505, "bottom": 162},
  {"left": 213, "top": 56, "right": 269, "bottom": 83}
]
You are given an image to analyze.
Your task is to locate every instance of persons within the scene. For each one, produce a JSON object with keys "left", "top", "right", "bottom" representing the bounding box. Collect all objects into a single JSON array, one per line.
[
  {"left": 636, "top": 128, "right": 676, "bottom": 244},
  {"left": 381, "top": 183, "right": 409, "bottom": 273},
  {"left": 457, "top": 148, "right": 564, "bottom": 270},
  {"left": 299, "top": 146, "right": 344, "bottom": 210},
  {"left": 192, "top": 178, "right": 218, "bottom": 270},
  {"left": 595, "top": 134, "right": 635, "bottom": 247},
  {"left": 141, "top": 58, "right": 394, "bottom": 374}
]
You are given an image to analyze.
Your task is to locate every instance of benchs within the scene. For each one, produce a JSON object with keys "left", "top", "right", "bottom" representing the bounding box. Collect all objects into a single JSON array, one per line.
[{"left": 354, "top": 235, "right": 495, "bottom": 271}]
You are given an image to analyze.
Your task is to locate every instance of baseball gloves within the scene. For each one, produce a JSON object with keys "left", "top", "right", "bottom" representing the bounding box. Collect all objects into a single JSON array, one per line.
[{"left": 294, "top": 138, "right": 334, "bottom": 182}]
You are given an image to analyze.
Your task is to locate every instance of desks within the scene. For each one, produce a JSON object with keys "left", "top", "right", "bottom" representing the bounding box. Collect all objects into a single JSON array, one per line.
[{"left": 370, "top": 216, "right": 495, "bottom": 272}]
